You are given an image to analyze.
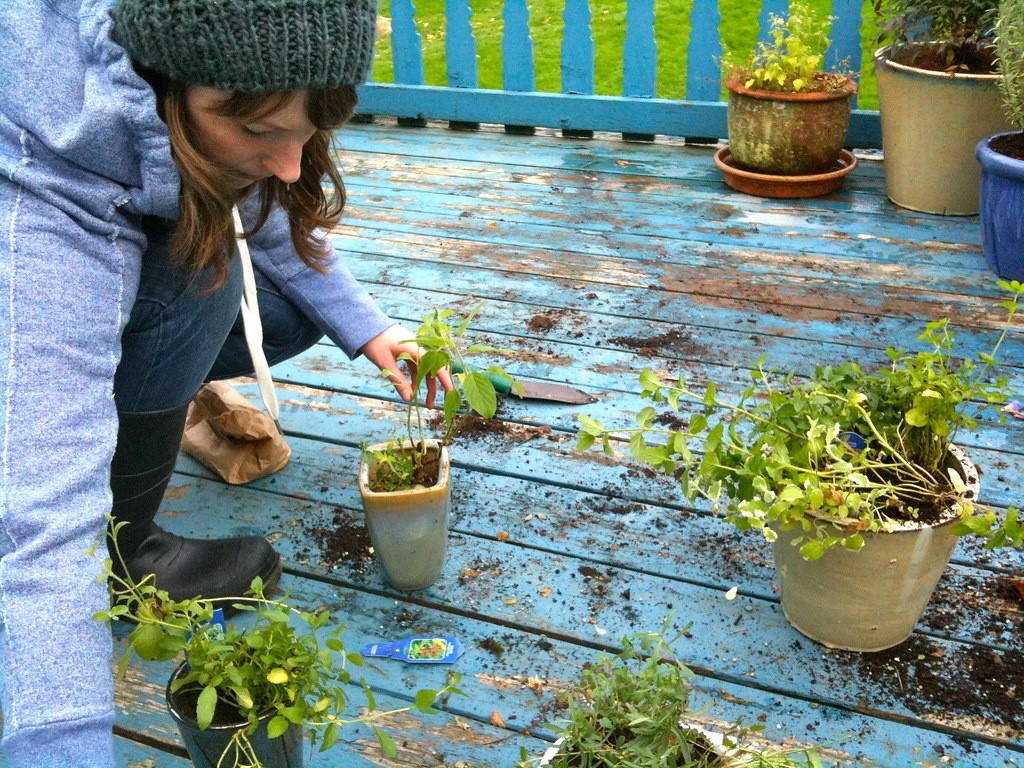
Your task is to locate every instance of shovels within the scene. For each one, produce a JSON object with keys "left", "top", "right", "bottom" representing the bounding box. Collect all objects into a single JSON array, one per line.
[{"left": 449, "top": 359, "right": 599, "bottom": 405}]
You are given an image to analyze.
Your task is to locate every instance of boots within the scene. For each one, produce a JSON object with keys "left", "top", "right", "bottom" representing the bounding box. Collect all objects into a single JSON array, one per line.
[{"left": 107, "top": 393, "right": 281, "bottom": 625}]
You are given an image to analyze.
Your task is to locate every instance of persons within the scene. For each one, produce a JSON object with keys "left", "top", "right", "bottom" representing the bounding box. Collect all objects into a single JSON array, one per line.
[{"left": 0, "top": 0, "right": 451, "bottom": 768}]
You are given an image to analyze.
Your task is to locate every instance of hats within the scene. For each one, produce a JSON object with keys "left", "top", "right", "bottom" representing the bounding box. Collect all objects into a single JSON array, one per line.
[{"left": 108, "top": 0, "right": 377, "bottom": 94}]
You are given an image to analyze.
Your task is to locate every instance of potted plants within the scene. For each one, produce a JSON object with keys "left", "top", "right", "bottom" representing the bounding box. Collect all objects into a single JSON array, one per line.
[
  {"left": 871, "top": 0, "right": 1024, "bottom": 216},
  {"left": 582, "top": 283, "right": 1024, "bottom": 643},
  {"left": 977, "top": 0, "right": 1024, "bottom": 281},
  {"left": 353, "top": 303, "right": 514, "bottom": 589},
  {"left": 511, "top": 623, "right": 851, "bottom": 768},
  {"left": 728, "top": 0, "right": 856, "bottom": 175},
  {"left": 94, "top": 514, "right": 467, "bottom": 768}
]
